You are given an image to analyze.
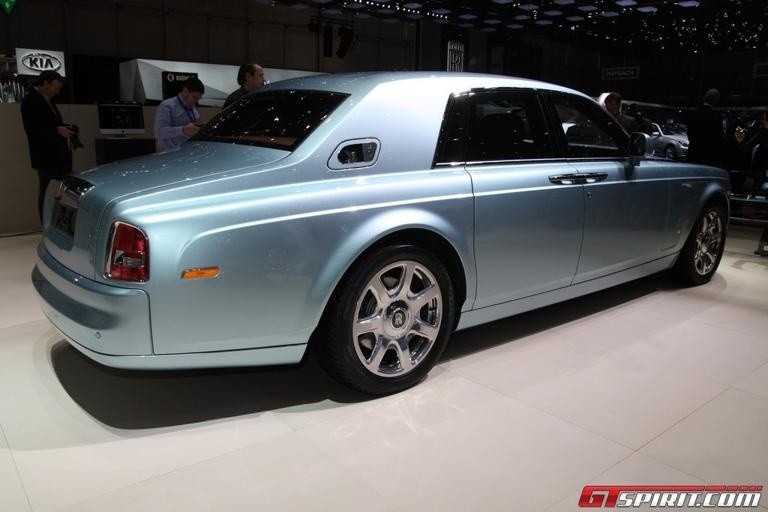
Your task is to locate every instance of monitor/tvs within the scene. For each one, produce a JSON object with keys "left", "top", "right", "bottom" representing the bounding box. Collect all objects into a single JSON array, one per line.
[{"left": 97, "top": 102, "right": 146, "bottom": 140}]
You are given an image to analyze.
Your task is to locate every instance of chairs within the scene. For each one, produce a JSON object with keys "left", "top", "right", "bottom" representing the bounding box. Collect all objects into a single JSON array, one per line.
[{"left": 462, "top": 112, "right": 527, "bottom": 163}]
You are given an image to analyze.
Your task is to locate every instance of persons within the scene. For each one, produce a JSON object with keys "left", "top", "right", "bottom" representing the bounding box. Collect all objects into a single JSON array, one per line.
[
  {"left": 20, "top": 69, "right": 78, "bottom": 228},
  {"left": 151, "top": 76, "right": 204, "bottom": 153},
  {"left": 603, "top": 91, "right": 636, "bottom": 135},
  {"left": 220, "top": 57, "right": 266, "bottom": 111},
  {"left": 684, "top": 88, "right": 732, "bottom": 173}
]
